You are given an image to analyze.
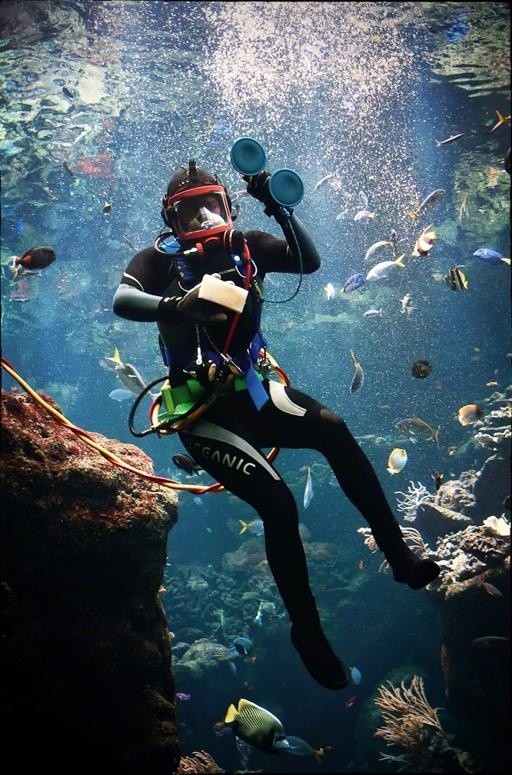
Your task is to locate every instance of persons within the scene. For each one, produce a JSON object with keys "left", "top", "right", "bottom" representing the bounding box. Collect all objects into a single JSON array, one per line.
[{"left": 113, "top": 155, "right": 444, "bottom": 692}]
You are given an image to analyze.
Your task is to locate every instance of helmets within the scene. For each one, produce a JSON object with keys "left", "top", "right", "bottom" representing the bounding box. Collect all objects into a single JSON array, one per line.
[{"left": 161, "top": 161, "right": 237, "bottom": 253}]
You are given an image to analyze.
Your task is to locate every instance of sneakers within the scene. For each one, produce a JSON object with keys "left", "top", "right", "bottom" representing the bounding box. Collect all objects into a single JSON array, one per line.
[
  {"left": 392, "top": 553, "right": 439, "bottom": 591},
  {"left": 290, "top": 619, "right": 347, "bottom": 688}
]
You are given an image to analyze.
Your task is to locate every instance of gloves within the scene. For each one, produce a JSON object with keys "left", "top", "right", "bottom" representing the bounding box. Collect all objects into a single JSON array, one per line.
[
  {"left": 246, "top": 171, "right": 294, "bottom": 222},
  {"left": 159, "top": 275, "right": 236, "bottom": 324}
]
